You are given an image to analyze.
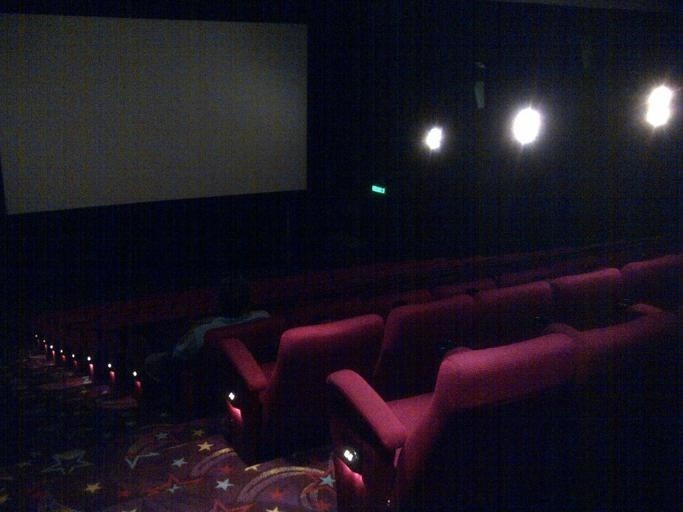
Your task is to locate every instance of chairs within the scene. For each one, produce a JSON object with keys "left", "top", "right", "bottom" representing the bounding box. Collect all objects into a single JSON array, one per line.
[{"left": 30, "top": 236, "right": 682, "bottom": 512}]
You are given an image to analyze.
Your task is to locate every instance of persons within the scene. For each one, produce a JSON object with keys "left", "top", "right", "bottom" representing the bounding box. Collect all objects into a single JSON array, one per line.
[{"left": 142, "top": 278, "right": 271, "bottom": 384}]
